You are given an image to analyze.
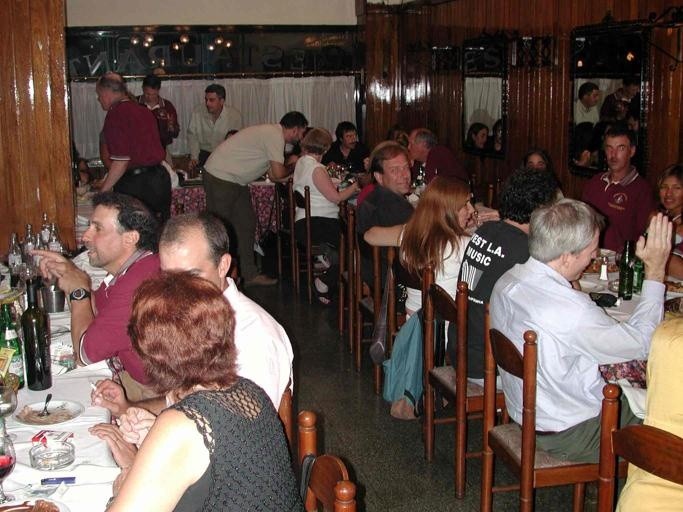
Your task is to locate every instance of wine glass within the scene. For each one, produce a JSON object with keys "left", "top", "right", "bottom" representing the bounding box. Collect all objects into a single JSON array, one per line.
[
  {"left": 0, "top": 385, "right": 18, "bottom": 437},
  {"left": 0, "top": 438, "right": 16, "bottom": 504}
]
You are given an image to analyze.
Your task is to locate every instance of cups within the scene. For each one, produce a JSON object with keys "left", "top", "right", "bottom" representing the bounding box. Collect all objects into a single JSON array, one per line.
[{"left": 41, "top": 286, "right": 66, "bottom": 313}]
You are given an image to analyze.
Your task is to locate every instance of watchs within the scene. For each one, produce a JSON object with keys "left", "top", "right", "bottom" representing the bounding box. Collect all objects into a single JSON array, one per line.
[{"left": 69, "top": 288, "right": 90, "bottom": 303}]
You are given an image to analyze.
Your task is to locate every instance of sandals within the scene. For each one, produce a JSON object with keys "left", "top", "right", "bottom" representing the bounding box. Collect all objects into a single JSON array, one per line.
[{"left": 311, "top": 276, "right": 332, "bottom": 305}]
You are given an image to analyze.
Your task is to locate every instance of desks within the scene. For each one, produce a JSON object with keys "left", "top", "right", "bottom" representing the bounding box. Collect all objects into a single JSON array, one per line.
[{"left": 162, "top": 166, "right": 283, "bottom": 275}]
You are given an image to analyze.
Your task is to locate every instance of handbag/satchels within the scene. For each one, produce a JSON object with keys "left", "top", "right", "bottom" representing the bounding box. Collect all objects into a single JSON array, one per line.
[{"left": 369, "top": 288, "right": 389, "bottom": 364}]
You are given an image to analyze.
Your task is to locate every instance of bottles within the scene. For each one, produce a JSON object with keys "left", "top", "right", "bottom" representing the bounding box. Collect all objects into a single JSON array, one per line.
[
  {"left": 467, "top": 174, "right": 503, "bottom": 211},
  {"left": 189, "top": 160, "right": 197, "bottom": 179},
  {"left": 598, "top": 257, "right": 609, "bottom": 281},
  {"left": 410, "top": 161, "right": 427, "bottom": 187},
  {"left": 336, "top": 162, "right": 353, "bottom": 181},
  {"left": 22, "top": 278, "right": 52, "bottom": 391},
  {"left": 617, "top": 232, "right": 648, "bottom": 301},
  {"left": 0, "top": 302, "right": 25, "bottom": 391},
  {"left": 8, "top": 211, "right": 64, "bottom": 280}
]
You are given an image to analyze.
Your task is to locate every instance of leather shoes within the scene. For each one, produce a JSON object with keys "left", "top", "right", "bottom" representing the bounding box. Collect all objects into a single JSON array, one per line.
[{"left": 244, "top": 275, "right": 279, "bottom": 287}]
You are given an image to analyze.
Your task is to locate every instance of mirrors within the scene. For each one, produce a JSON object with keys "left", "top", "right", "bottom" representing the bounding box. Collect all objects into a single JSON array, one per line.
[
  {"left": 565, "top": 19, "right": 653, "bottom": 179},
  {"left": 460, "top": 32, "right": 510, "bottom": 161}
]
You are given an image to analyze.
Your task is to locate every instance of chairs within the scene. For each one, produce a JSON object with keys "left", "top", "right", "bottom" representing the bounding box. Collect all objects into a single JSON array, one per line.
[
  {"left": 266, "top": 178, "right": 403, "bottom": 398},
  {"left": 595, "top": 382, "right": 682, "bottom": 512},
  {"left": 468, "top": 296, "right": 629, "bottom": 512},
  {"left": 271, "top": 386, "right": 318, "bottom": 469},
  {"left": 295, "top": 449, "right": 365, "bottom": 512},
  {"left": 415, "top": 262, "right": 509, "bottom": 501}
]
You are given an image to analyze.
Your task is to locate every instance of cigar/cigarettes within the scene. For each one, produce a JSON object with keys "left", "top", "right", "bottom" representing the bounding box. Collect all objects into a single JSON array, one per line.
[{"left": 88, "top": 382, "right": 102, "bottom": 396}]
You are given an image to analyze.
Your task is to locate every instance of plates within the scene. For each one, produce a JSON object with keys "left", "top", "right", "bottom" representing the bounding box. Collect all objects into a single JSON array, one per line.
[
  {"left": 12, "top": 399, "right": 85, "bottom": 428},
  {"left": 0, "top": 496, "right": 71, "bottom": 512}
]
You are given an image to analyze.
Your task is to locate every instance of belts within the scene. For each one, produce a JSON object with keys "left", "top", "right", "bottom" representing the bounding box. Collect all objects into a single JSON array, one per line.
[{"left": 126, "top": 164, "right": 161, "bottom": 176}]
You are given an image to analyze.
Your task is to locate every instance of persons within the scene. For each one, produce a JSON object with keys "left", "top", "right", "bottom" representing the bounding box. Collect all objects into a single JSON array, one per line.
[
  {"left": 616, "top": 315, "right": 683, "bottom": 512},
  {"left": 573, "top": 74, "right": 640, "bottom": 129},
  {"left": 88, "top": 211, "right": 295, "bottom": 471},
  {"left": 184, "top": 84, "right": 243, "bottom": 172},
  {"left": 72, "top": 141, "right": 92, "bottom": 188},
  {"left": 577, "top": 125, "right": 658, "bottom": 255},
  {"left": 88, "top": 72, "right": 172, "bottom": 226},
  {"left": 292, "top": 120, "right": 565, "bottom": 388},
  {"left": 133, "top": 74, "right": 179, "bottom": 166},
  {"left": 647, "top": 161, "right": 682, "bottom": 293},
  {"left": 102, "top": 268, "right": 308, "bottom": 512},
  {"left": 198, "top": 110, "right": 307, "bottom": 289},
  {"left": 487, "top": 198, "right": 672, "bottom": 467},
  {"left": 224, "top": 130, "right": 238, "bottom": 142},
  {"left": 29, "top": 191, "right": 166, "bottom": 403}
]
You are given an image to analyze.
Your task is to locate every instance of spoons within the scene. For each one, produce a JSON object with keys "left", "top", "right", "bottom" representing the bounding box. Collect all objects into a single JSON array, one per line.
[{"left": 35, "top": 394, "right": 52, "bottom": 420}]
